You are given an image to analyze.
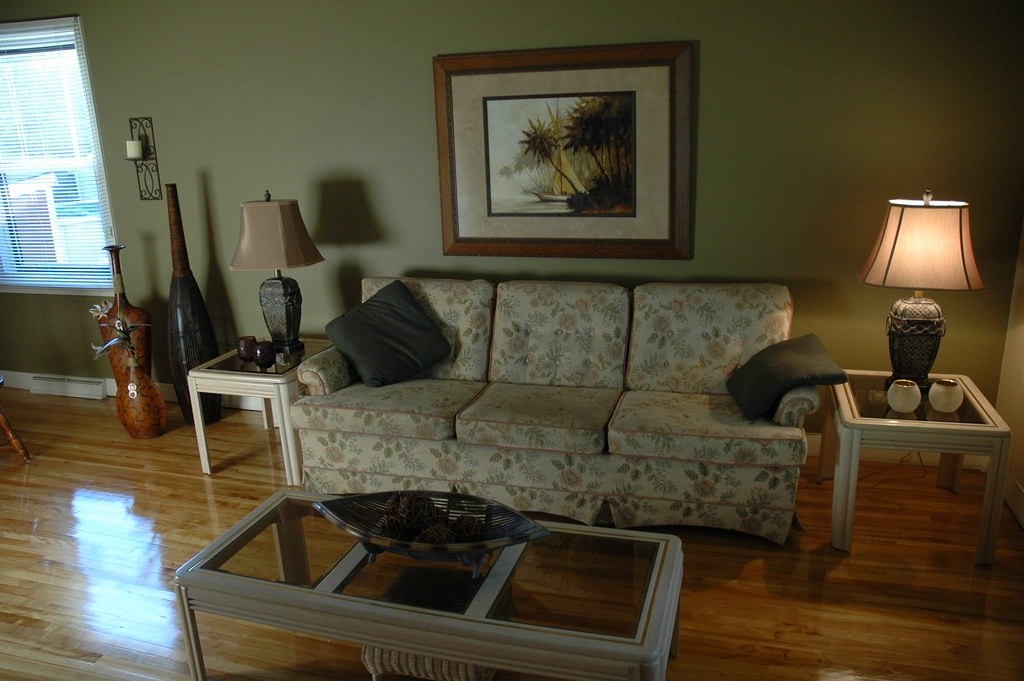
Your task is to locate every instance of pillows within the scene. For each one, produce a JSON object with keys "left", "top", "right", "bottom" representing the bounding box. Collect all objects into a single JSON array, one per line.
[
  {"left": 726, "top": 333, "right": 848, "bottom": 421},
  {"left": 325, "top": 280, "right": 451, "bottom": 387}
]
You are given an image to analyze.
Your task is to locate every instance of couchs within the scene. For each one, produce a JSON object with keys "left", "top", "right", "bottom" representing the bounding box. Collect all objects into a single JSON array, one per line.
[{"left": 288, "top": 278, "right": 822, "bottom": 545}]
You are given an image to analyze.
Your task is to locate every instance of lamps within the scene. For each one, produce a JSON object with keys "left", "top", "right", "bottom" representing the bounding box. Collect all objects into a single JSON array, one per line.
[
  {"left": 229, "top": 189, "right": 326, "bottom": 354},
  {"left": 859, "top": 189, "right": 986, "bottom": 392}
]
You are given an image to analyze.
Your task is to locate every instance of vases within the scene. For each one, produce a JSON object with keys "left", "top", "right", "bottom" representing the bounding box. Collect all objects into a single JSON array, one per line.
[
  {"left": 166, "top": 184, "right": 222, "bottom": 427},
  {"left": 116, "top": 358, "right": 168, "bottom": 438},
  {"left": 96, "top": 244, "right": 151, "bottom": 386}
]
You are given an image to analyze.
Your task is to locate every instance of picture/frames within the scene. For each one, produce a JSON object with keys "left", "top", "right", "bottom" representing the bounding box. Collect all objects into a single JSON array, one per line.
[{"left": 432, "top": 42, "right": 695, "bottom": 260}]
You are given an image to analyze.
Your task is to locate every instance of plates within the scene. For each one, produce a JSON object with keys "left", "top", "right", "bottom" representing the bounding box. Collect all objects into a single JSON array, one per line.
[{"left": 312, "top": 489, "right": 552, "bottom": 578}]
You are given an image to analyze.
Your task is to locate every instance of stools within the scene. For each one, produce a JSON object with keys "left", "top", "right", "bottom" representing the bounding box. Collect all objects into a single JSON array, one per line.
[{"left": 361, "top": 566, "right": 511, "bottom": 680}]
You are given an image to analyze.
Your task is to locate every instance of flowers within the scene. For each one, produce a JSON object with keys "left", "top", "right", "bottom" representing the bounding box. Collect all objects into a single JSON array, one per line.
[{"left": 89, "top": 300, "right": 153, "bottom": 361}]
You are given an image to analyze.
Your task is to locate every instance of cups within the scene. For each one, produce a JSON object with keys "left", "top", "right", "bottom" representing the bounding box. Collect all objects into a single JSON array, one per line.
[
  {"left": 237, "top": 336, "right": 257, "bottom": 362},
  {"left": 928, "top": 379, "right": 964, "bottom": 412},
  {"left": 887, "top": 380, "right": 921, "bottom": 412},
  {"left": 254, "top": 341, "right": 275, "bottom": 368}
]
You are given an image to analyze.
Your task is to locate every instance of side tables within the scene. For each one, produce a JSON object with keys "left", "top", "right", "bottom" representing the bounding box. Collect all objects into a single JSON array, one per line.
[
  {"left": 186, "top": 338, "right": 335, "bottom": 486},
  {"left": 817, "top": 369, "right": 1013, "bottom": 566}
]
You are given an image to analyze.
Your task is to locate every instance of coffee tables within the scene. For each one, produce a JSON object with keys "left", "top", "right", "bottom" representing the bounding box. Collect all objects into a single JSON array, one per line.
[{"left": 173, "top": 488, "right": 684, "bottom": 681}]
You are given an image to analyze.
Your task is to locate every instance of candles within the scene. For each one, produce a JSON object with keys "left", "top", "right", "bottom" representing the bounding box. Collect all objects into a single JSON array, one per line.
[{"left": 127, "top": 140, "right": 143, "bottom": 159}]
